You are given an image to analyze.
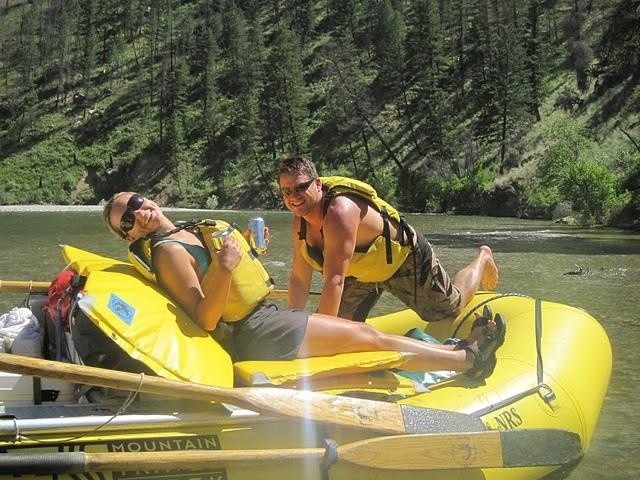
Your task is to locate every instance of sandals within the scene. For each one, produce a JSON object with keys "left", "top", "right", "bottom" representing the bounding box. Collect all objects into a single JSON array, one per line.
[{"left": 456, "top": 304, "right": 507, "bottom": 381}]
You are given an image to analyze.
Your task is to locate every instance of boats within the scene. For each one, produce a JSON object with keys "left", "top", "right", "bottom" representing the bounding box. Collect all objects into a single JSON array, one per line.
[{"left": 1, "top": 288, "right": 613, "bottom": 479}]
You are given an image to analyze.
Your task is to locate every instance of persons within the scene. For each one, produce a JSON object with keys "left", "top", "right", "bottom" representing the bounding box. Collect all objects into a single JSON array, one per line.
[
  {"left": 272, "top": 157, "right": 499, "bottom": 322},
  {"left": 101, "top": 191, "right": 504, "bottom": 379}
]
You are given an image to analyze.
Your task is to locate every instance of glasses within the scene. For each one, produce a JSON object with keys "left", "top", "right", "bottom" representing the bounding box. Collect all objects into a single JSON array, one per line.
[
  {"left": 120, "top": 195, "right": 144, "bottom": 239},
  {"left": 281, "top": 178, "right": 315, "bottom": 197}
]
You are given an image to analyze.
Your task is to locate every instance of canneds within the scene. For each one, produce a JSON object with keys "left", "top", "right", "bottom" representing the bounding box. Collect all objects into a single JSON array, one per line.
[
  {"left": 211, "top": 228, "right": 231, "bottom": 250},
  {"left": 249, "top": 216, "right": 267, "bottom": 255}
]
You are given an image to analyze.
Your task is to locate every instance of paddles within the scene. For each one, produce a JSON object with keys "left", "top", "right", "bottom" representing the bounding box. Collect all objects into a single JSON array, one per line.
[
  {"left": 0, "top": 352, "right": 485, "bottom": 435},
  {"left": 0, "top": 280, "right": 323, "bottom": 300},
  {"left": 0, "top": 428, "right": 584, "bottom": 473}
]
